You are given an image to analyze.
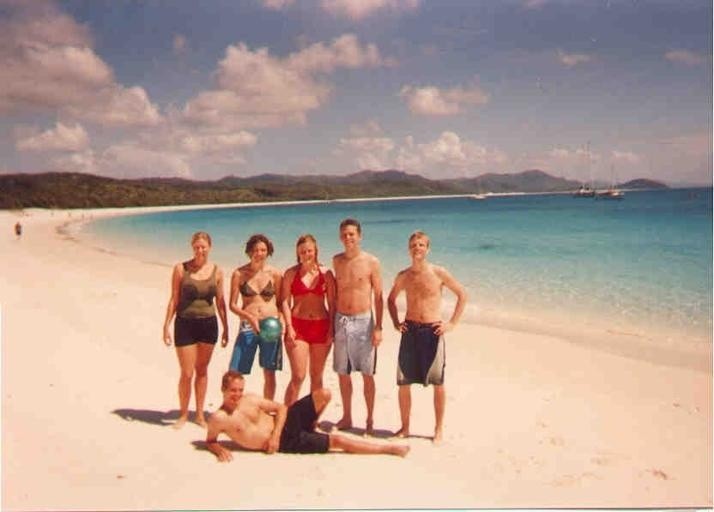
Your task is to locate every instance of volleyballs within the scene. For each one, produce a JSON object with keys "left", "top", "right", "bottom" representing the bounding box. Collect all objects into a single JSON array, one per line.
[{"left": 258, "top": 317, "right": 282, "bottom": 343}]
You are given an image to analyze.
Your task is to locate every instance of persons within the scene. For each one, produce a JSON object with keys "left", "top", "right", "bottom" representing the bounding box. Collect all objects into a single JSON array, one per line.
[
  {"left": 163, "top": 231, "right": 230, "bottom": 430},
  {"left": 205, "top": 371, "right": 411, "bottom": 464},
  {"left": 227, "top": 234, "right": 284, "bottom": 401},
  {"left": 330, "top": 217, "right": 385, "bottom": 436},
  {"left": 15, "top": 221, "right": 22, "bottom": 241},
  {"left": 280, "top": 233, "right": 338, "bottom": 407},
  {"left": 386, "top": 231, "right": 468, "bottom": 445}
]
